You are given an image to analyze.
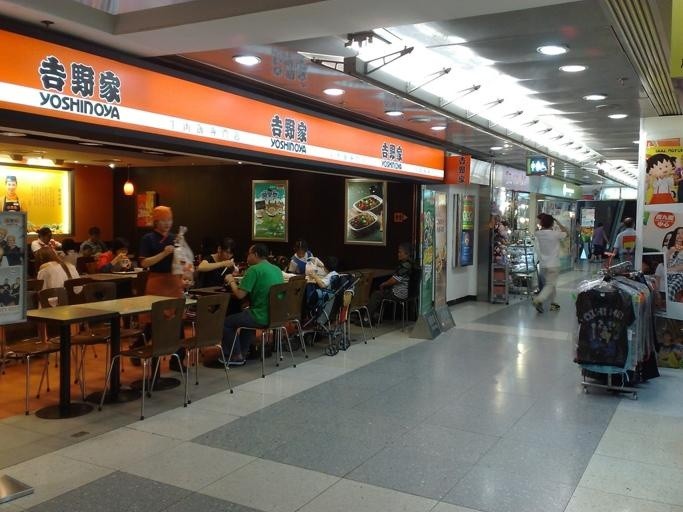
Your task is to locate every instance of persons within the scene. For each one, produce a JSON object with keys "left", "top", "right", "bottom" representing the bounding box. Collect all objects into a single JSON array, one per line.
[
  {"left": 32, "top": 249, "right": 83, "bottom": 311},
  {"left": 80, "top": 228, "right": 106, "bottom": 256},
  {"left": 655, "top": 332, "right": 676, "bottom": 367},
  {"left": 194, "top": 237, "right": 238, "bottom": 286},
  {"left": 77, "top": 246, "right": 93, "bottom": 276},
  {"left": 128, "top": 205, "right": 190, "bottom": 374},
  {"left": 1, "top": 175, "right": 25, "bottom": 212},
  {"left": 0, "top": 277, "right": 20, "bottom": 307},
  {"left": 218, "top": 242, "right": 284, "bottom": 364},
  {"left": 0, "top": 228, "right": 24, "bottom": 267},
  {"left": 667, "top": 226, "right": 683, "bottom": 302},
  {"left": 527, "top": 213, "right": 569, "bottom": 313},
  {"left": 355, "top": 243, "right": 413, "bottom": 327},
  {"left": 310, "top": 258, "right": 340, "bottom": 291},
  {"left": 662, "top": 232, "right": 674, "bottom": 246},
  {"left": 610, "top": 218, "right": 636, "bottom": 270},
  {"left": 30, "top": 228, "right": 62, "bottom": 261},
  {"left": 591, "top": 222, "right": 610, "bottom": 263},
  {"left": 288, "top": 239, "right": 316, "bottom": 277},
  {"left": 62, "top": 239, "right": 80, "bottom": 267},
  {"left": 653, "top": 262, "right": 666, "bottom": 312},
  {"left": 97, "top": 237, "right": 133, "bottom": 272}
]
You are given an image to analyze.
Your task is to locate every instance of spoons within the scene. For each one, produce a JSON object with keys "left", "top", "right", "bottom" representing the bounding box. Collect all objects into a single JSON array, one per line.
[{"left": 233, "top": 264, "right": 239, "bottom": 272}]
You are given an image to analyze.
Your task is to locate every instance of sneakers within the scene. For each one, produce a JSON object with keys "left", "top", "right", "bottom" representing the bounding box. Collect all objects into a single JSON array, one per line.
[
  {"left": 169, "top": 362, "right": 186, "bottom": 372},
  {"left": 218, "top": 354, "right": 246, "bottom": 365},
  {"left": 549, "top": 303, "right": 561, "bottom": 311},
  {"left": 355, "top": 318, "right": 374, "bottom": 327},
  {"left": 531, "top": 300, "right": 545, "bottom": 313},
  {"left": 129, "top": 346, "right": 141, "bottom": 366}
]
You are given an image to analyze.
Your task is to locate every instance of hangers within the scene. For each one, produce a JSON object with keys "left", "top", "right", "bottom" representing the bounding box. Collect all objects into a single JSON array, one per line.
[{"left": 598, "top": 259, "right": 634, "bottom": 282}]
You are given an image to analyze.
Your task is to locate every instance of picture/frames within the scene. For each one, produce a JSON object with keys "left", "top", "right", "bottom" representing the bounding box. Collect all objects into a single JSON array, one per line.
[
  {"left": 344, "top": 178, "right": 387, "bottom": 247},
  {"left": 251, "top": 178, "right": 288, "bottom": 243}
]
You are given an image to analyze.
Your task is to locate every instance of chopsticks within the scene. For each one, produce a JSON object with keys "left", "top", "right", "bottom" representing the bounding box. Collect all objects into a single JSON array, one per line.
[{"left": 221, "top": 258, "right": 234, "bottom": 276}]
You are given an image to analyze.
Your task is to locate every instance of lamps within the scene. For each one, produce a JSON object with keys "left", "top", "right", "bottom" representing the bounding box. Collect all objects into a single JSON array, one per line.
[{"left": 123, "top": 164, "right": 135, "bottom": 194}]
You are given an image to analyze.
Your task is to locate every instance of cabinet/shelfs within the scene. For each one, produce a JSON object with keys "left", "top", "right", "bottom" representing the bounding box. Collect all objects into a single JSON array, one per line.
[
  {"left": 512, "top": 226, "right": 538, "bottom": 299},
  {"left": 491, "top": 204, "right": 513, "bottom": 305}
]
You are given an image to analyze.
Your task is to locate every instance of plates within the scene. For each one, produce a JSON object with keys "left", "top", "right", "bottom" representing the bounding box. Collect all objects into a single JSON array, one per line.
[
  {"left": 353, "top": 195, "right": 382, "bottom": 213},
  {"left": 348, "top": 211, "right": 378, "bottom": 232}
]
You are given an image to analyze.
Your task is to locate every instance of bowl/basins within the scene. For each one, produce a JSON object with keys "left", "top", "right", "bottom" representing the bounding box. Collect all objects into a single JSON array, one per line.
[
  {"left": 134, "top": 267, "right": 143, "bottom": 271},
  {"left": 233, "top": 276, "right": 243, "bottom": 282},
  {"left": 120, "top": 259, "right": 129, "bottom": 268}
]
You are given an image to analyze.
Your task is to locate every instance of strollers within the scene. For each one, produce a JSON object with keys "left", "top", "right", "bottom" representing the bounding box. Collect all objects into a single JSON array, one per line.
[{"left": 301, "top": 271, "right": 363, "bottom": 356}]
